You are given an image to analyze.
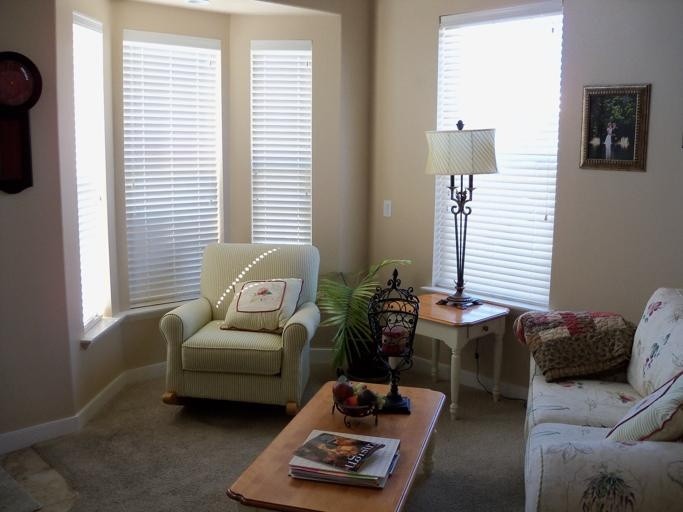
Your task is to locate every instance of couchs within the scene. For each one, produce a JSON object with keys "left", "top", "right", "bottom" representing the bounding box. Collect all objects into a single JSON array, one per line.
[{"left": 511, "top": 283, "right": 683, "bottom": 510}]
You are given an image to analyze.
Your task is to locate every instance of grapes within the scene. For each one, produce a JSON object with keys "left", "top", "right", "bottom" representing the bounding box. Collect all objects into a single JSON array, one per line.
[{"left": 353, "top": 383, "right": 386, "bottom": 410}]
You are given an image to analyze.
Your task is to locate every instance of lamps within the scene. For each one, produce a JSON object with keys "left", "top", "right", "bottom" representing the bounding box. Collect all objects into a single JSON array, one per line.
[{"left": 422, "top": 119, "right": 502, "bottom": 311}]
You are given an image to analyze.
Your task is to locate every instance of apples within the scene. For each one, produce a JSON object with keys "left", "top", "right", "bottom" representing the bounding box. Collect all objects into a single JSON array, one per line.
[{"left": 332, "top": 381, "right": 374, "bottom": 407}]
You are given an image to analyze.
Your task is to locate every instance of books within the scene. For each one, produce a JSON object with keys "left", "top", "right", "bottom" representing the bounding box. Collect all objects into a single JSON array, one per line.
[{"left": 288, "top": 429, "right": 402, "bottom": 490}]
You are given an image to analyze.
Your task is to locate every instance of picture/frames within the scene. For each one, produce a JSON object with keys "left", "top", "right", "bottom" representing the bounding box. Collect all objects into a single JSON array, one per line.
[{"left": 577, "top": 81, "right": 651, "bottom": 174}]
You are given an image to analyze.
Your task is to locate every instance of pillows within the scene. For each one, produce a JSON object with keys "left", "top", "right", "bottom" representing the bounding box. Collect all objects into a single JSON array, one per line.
[
  {"left": 606, "top": 371, "right": 682, "bottom": 444},
  {"left": 218, "top": 276, "right": 302, "bottom": 337}
]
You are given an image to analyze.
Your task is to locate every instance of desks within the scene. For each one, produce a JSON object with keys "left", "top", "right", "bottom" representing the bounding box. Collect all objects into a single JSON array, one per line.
[{"left": 389, "top": 292, "right": 509, "bottom": 417}]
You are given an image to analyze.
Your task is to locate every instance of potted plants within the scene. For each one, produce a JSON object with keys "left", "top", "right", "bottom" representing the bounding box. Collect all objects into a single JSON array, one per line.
[{"left": 314, "top": 252, "right": 416, "bottom": 383}]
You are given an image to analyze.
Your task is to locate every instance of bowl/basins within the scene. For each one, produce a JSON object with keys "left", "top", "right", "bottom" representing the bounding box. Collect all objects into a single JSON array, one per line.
[{"left": 333, "top": 396, "right": 371, "bottom": 415}]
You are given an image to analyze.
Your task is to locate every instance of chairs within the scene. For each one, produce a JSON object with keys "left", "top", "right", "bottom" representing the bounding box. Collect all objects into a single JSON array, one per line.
[{"left": 155, "top": 235, "right": 324, "bottom": 418}]
